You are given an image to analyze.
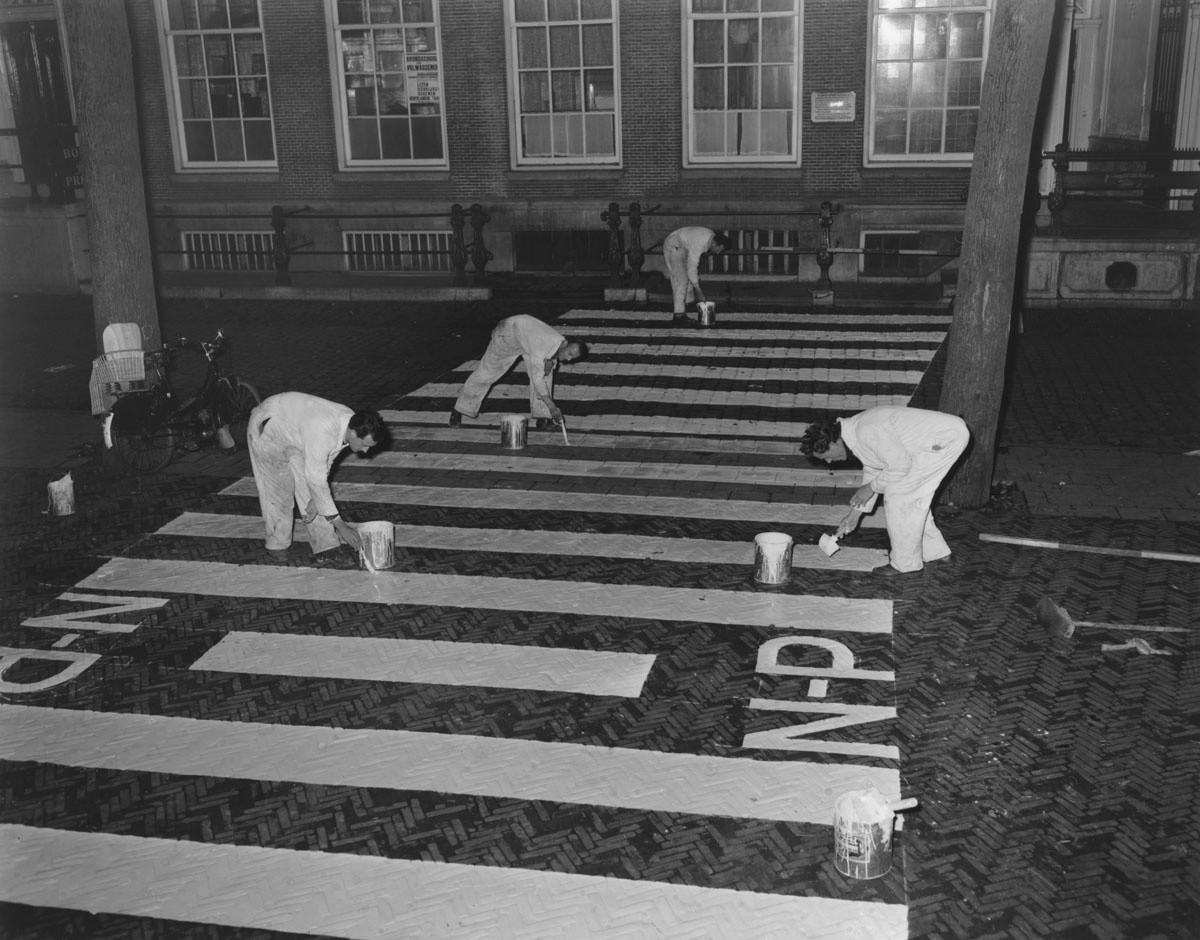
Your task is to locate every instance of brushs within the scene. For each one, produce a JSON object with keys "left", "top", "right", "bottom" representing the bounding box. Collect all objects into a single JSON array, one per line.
[{"left": 818, "top": 533, "right": 840, "bottom": 557}]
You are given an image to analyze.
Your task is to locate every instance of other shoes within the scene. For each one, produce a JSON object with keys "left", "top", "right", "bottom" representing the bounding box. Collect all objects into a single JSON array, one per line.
[
  {"left": 266, "top": 548, "right": 294, "bottom": 563},
  {"left": 449, "top": 408, "right": 462, "bottom": 428},
  {"left": 872, "top": 563, "right": 925, "bottom": 581},
  {"left": 310, "top": 547, "right": 355, "bottom": 567},
  {"left": 536, "top": 418, "right": 563, "bottom": 432},
  {"left": 922, "top": 552, "right": 955, "bottom": 569},
  {"left": 672, "top": 314, "right": 696, "bottom": 324},
  {"left": 685, "top": 302, "right": 697, "bottom": 313}
]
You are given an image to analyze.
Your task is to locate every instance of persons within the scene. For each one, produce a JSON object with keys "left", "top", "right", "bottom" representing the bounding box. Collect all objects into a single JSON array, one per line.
[
  {"left": 247, "top": 391, "right": 391, "bottom": 568},
  {"left": 662, "top": 225, "right": 731, "bottom": 326},
  {"left": 447, "top": 314, "right": 589, "bottom": 432},
  {"left": 799, "top": 405, "right": 970, "bottom": 578}
]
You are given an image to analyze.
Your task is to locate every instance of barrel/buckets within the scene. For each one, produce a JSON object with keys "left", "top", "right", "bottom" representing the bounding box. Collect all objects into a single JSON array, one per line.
[
  {"left": 697, "top": 301, "right": 716, "bottom": 325},
  {"left": 357, "top": 521, "right": 395, "bottom": 569},
  {"left": 835, "top": 791, "right": 894, "bottom": 880},
  {"left": 51, "top": 482, "right": 76, "bottom": 516},
  {"left": 754, "top": 531, "right": 793, "bottom": 585},
  {"left": 500, "top": 415, "right": 528, "bottom": 450}
]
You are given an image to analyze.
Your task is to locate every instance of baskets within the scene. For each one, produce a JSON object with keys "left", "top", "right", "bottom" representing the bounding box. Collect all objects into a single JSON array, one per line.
[{"left": 91, "top": 349, "right": 147, "bottom": 382}]
